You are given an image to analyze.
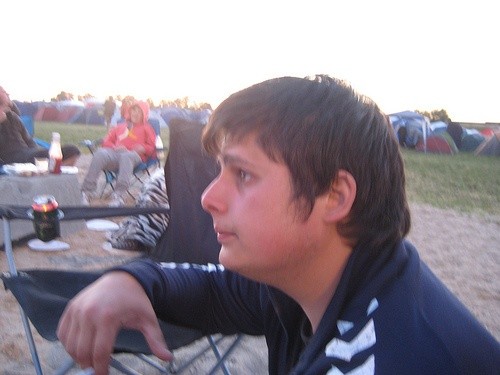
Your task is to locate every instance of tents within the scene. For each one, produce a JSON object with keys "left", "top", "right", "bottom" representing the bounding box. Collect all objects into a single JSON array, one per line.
[
  {"left": 148, "top": 106, "right": 213, "bottom": 134},
  {"left": 11, "top": 98, "right": 123, "bottom": 126},
  {"left": 386, "top": 111, "right": 500, "bottom": 155}
]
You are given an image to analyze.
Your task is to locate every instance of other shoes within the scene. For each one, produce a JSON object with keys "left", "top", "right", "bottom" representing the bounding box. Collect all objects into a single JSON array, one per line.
[
  {"left": 108, "top": 198, "right": 124, "bottom": 209},
  {"left": 81, "top": 192, "right": 89, "bottom": 205}
]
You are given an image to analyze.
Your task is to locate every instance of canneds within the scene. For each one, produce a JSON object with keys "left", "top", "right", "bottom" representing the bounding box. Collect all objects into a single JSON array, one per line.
[{"left": 32, "top": 194, "right": 61, "bottom": 243}]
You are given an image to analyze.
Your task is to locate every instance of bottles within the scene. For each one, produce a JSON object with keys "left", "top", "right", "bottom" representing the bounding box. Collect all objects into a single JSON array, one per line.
[{"left": 48, "top": 132, "right": 63, "bottom": 174}]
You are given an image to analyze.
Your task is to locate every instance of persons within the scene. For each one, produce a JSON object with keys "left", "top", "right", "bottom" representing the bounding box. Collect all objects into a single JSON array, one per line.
[
  {"left": 58, "top": 74, "right": 500, "bottom": 375},
  {"left": 80, "top": 100, "right": 159, "bottom": 208},
  {"left": 446, "top": 118, "right": 463, "bottom": 148},
  {"left": 103, "top": 96, "right": 116, "bottom": 131},
  {"left": 0, "top": 105, "right": 81, "bottom": 167},
  {"left": 397, "top": 125, "right": 408, "bottom": 148},
  {"left": 0, "top": 86, "right": 21, "bottom": 116}
]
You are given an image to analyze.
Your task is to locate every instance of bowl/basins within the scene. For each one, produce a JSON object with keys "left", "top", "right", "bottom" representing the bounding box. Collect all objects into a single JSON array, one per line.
[{"left": 34, "top": 157, "right": 48, "bottom": 171}]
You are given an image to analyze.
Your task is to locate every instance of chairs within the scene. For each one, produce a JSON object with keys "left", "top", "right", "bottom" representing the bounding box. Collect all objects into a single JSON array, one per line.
[{"left": 0, "top": 118, "right": 243, "bottom": 375}]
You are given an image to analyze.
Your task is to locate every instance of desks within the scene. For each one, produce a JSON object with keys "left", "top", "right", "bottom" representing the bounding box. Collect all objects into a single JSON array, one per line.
[{"left": 0, "top": 173, "right": 88, "bottom": 247}]
